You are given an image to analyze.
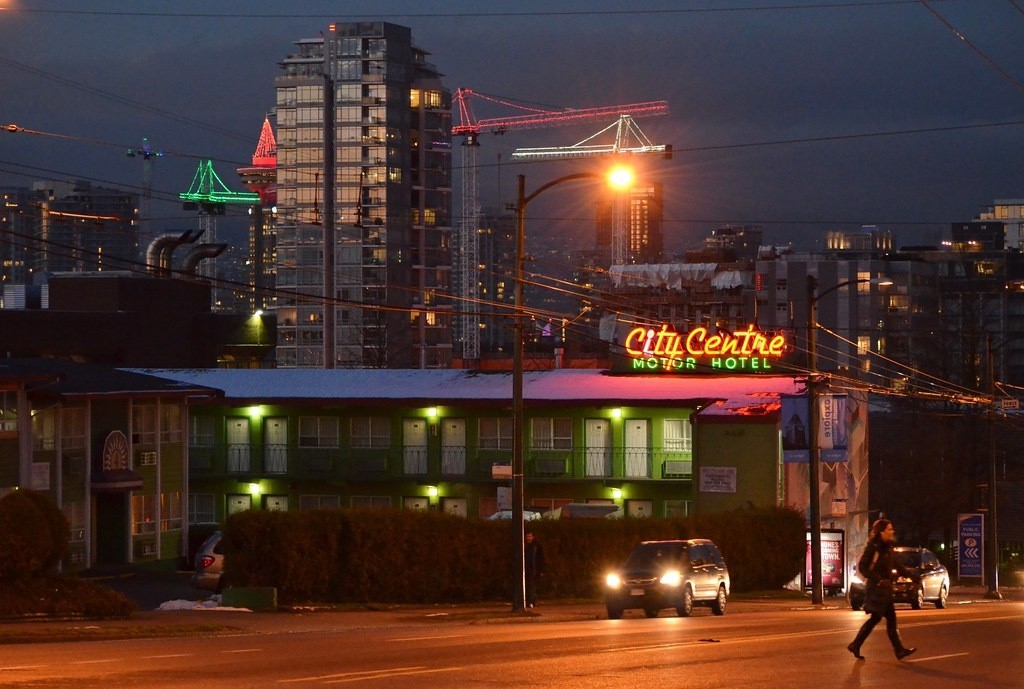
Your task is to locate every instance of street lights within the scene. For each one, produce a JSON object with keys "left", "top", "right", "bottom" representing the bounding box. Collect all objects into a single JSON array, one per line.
[{"left": 508, "top": 161, "right": 644, "bottom": 603}]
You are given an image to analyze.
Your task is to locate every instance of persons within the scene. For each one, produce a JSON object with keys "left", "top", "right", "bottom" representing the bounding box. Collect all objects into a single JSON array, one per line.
[
  {"left": 524, "top": 531, "right": 545, "bottom": 608},
  {"left": 846, "top": 519, "right": 930, "bottom": 660}
]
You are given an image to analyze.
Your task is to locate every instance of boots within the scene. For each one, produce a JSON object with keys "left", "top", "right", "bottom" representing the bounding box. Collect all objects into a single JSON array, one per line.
[
  {"left": 848, "top": 622, "right": 875, "bottom": 660},
  {"left": 886, "top": 627, "right": 918, "bottom": 661}
]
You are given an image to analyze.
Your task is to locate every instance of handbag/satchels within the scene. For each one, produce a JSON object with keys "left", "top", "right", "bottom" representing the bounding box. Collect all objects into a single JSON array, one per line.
[{"left": 855, "top": 552, "right": 868, "bottom": 585}]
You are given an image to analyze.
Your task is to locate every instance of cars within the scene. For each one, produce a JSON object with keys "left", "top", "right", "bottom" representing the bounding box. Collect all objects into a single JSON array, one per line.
[
  {"left": 849, "top": 546, "right": 952, "bottom": 610},
  {"left": 191, "top": 530, "right": 226, "bottom": 590}
]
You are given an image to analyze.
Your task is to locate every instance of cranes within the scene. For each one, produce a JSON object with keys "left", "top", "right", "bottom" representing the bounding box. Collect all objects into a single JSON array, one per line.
[
  {"left": 446, "top": 86, "right": 679, "bottom": 360},
  {"left": 128, "top": 134, "right": 216, "bottom": 258},
  {"left": 180, "top": 159, "right": 262, "bottom": 313},
  {"left": 508, "top": 112, "right": 673, "bottom": 267}
]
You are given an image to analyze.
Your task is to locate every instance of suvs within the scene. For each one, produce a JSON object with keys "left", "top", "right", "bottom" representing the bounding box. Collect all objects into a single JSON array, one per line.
[{"left": 607, "top": 538, "right": 731, "bottom": 616}]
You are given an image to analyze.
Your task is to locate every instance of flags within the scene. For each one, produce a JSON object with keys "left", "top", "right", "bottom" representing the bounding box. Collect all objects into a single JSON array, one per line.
[{"left": 599, "top": 315, "right": 616, "bottom": 342}]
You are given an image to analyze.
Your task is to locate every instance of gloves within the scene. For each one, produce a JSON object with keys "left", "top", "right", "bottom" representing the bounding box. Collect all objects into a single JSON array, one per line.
[{"left": 881, "top": 579, "right": 894, "bottom": 595}]
